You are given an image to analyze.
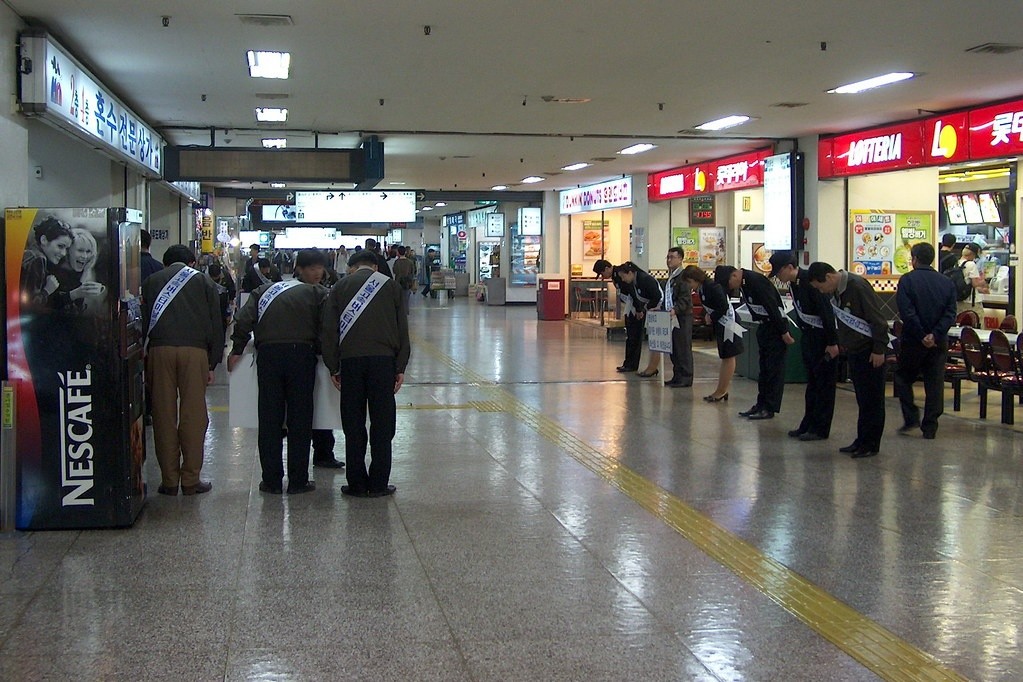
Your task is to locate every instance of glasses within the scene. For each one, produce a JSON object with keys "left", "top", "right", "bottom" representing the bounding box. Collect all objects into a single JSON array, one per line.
[{"left": 667, "top": 255, "right": 681, "bottom": 259}]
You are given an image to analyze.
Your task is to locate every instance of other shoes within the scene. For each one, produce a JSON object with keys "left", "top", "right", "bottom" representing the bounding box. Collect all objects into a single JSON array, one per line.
[
  {"left": 316, "top": 458, "right": 345, "bottom": 468},
  {"left": 800, "top": 432, "right": 822, "bottom": 440},
  {"left": 421, "top": 292, "right": 427, "bottom": 297},
  {"left": 158, "top": 486, "right": 178, "bottom": 496},
  {"left": 369, "top": 485, "right": 396, "bottom": 498},
  {"left": 788, "top": 429, "right": 806, "bottom": 437},
  {"left": 749, "top": 408, "right": 774, "bottom": 418},
  {"left": 181, "top": 482, "right": 212, "bottom": 495},
  {"left": 669, "top": 382, "right": 692, "bottom": 387},
  {"left": 341, "top": 486, "right": 368, "bottom": 497},
  {"left": 259, "top": 481, "right": 282, "bottom": 494},
  {"left": 617, "top": 366, "right": 636, "bottom": 371},
  {"left": 839, "top": 444, "right": 857, "bottom": 452},
  {"left": 288, "top": 482, "right": 315, "bottom": 494},
  {"left": 852, "top": 448, "right": 873, "bottom": 458},
  {"left": 923, "top": 430, "right": 935, "bottom": 439},
  {"left": 738, "top": 405, "right": 759, "bottom": 416},
  {"left": 899, "top": 420, "right": 920, "bottom": 433},
  {"left": 665, "top": 379, "right": 676, "bottom": 385}
]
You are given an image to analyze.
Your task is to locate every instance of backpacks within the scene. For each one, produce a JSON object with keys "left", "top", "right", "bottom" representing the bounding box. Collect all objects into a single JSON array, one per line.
[{"left": 943, "top": 260, "right": 972, "bottom": 301}]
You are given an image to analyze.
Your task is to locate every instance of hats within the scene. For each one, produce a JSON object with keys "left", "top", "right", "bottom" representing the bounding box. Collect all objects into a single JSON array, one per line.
[
  {"left": 965, "top": 243, "right": 980, "bottom": 258},
  {"left": 593, "top": 259, "right": 607, "bottom": 280},
  {"left": 714, "top": 266, "right": 732, "bottom": 294},
  {"left": 768, "top": 253, "right": 790, "bottom": 278}
]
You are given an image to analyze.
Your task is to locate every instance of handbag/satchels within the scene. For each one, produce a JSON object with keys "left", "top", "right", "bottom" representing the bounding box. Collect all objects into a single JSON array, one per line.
[{"left": 400, "top": 278, "right": 412, "bottom": 290}]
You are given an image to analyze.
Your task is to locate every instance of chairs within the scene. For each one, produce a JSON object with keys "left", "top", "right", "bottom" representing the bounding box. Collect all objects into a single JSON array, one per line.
[
  {"left": 571, "top": 287, "right": 610, "bottom": 322},
  {"left": 861, "top": 305, "right": 1023, "bottom": 432}
]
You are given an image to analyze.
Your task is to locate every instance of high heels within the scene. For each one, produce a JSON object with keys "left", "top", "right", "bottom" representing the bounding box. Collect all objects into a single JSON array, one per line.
[
  {"left": 704, "top": 392, "right": 728, "bottom": 402},
  {"left": 639, "top": 369, "right": 658, "bottom": 378}
]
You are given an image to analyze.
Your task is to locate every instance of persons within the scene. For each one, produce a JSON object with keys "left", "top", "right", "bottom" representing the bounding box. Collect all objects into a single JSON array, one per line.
[
  {"left": 204, "top": 233, "right": 417, "bottom": 352},
  {"left": 226, "top": 280, "right": 320, "bottom": 496},
  {"left": 421, "top": 248, "right": 436, "bottom": 297},
  {"left": 295, "top": 247, "right": 346, "bottom": 473},
  {"left": 714, "top": 265, "right": 796, "bottom": 420},
  {"left": 938, "top": 233, "right": 958, "bottom": 274},
  {"left": 662, "top": 246, "right": 696, "bottom": 389},
  {"left": 47, "top": 226, "right": 100, "bottom": 316},
  {"left": 322, "top": 249, "right": 411, "bottom": 499},
  {"left": 139, "top": 228, "right": 166, "bottom": 425},
  {"left": 952, "top": 243, "right": 989, "bottom": 331},
  {"left": 895, "top": 241, "right": 958, "bottom": 440},
  {"left": 807, "top": 261, "right": 890, "bottom": 461},
  {"left": 141, "top": 244, "right": 224, "bottom": 498},
  {"left": 19, "top": 216, "right": 74, "bottom": 316},
  {"left": 767, "top": 251, "right": 840, "bottom": 442},
  {"left": 682, "top": 265, "right": 745, "bottom": 402},
  {"left": 971, "top": 234, "right": 988, "bottom": 281},
  {"left": 593, "top": 259, "right": 646, "bottom": 372},
  {"left": 616, "top": 260, "right": 666, "bottom": 378}
]
